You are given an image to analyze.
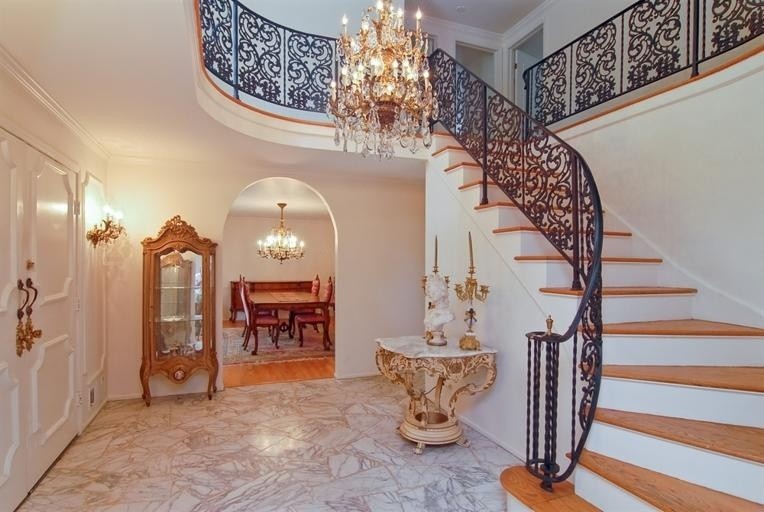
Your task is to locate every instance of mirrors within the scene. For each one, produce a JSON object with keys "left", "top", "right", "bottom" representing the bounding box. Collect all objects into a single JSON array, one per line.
[{"left": 141, "top": 215, "right": 219, "bottom": 407}]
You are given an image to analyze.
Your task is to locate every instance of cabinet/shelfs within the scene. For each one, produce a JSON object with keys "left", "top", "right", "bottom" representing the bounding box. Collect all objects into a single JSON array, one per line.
[
  {"left": 229, "top": 282, "right": 312, "bottom": 323},
  {"left": 0, "top": 119, "right": 108, "bottom": 512}
]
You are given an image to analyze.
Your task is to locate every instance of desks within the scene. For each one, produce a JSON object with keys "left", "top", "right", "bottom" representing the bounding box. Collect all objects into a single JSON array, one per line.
[{"left": 375, "top": 335, "right": 497, "bottom": 454}]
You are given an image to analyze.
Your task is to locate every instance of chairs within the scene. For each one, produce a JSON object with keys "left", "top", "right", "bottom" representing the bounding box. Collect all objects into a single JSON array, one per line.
[{"left": 240, "top": 273, "right": 335, "bottom": 355}]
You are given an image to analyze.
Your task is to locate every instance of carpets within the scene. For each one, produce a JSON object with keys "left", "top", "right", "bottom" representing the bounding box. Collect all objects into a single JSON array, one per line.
[{"left": 223, "top": 327, "right": 336, "bottom": 366}]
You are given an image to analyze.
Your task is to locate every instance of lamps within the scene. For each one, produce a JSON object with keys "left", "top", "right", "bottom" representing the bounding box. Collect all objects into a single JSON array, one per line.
[
  {"left": 326, "top": 0, "right": 439, "bottom": 162},
  {"left": 86, "top": 206, "right": 124, "bottom": 248},
  {"left": 256, "top": 203, "right": 304, "bottom": 262}
]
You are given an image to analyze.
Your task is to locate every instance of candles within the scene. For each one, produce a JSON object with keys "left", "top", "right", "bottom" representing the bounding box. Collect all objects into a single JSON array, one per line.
[
  {"left": 468, "top": 233, "right": 475, "bottom": 269},
  {"left": 434, "top": 236, "right": 438, "bottom": 270}
]
